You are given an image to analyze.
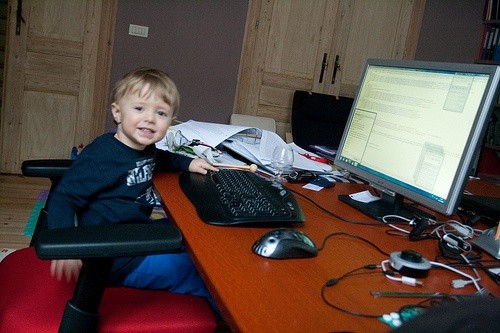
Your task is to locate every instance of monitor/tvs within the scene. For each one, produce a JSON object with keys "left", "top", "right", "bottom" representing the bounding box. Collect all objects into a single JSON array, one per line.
[{"left": 334, "top": 58, "right": 500, "bottom": 216}]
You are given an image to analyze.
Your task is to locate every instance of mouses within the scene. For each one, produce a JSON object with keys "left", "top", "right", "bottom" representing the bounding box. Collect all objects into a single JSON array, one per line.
[{"left": 252, "top": 229, "right": 318, "bottom": 259}]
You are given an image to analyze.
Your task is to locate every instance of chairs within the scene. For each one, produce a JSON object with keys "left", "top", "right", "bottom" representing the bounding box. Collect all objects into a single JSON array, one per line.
[
  {"left": 292, "top": 90, "right": 354, "bottom": 161},
  {"left": 0, "top": 159, "right": 217, "bottom": 333}
]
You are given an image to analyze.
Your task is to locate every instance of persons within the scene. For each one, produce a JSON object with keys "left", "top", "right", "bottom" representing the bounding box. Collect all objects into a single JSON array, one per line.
[{"left": 46, "top": 67, "right": 220, "bottom": 311}]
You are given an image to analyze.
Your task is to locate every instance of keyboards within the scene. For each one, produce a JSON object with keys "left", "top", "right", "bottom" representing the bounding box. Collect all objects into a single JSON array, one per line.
[{"left": 179, "top": 169, "right": 306, "bottom": 228}]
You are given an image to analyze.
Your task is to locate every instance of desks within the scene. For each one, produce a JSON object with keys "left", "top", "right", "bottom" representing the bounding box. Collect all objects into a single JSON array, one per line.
[{"left": 153, "top": 161, "right": 500, "bottom": 333}]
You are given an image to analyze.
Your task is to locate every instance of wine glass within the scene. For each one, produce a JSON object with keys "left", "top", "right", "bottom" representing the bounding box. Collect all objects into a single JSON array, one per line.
[{"left": 270, "top": 145, "right": 294, "bottom": 183}]
